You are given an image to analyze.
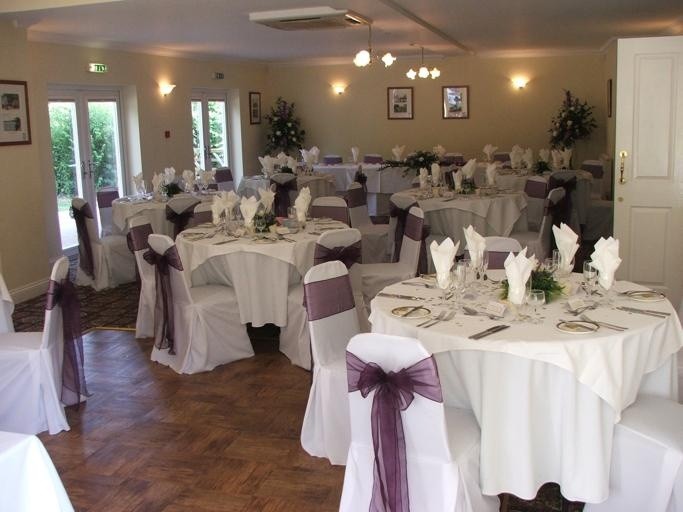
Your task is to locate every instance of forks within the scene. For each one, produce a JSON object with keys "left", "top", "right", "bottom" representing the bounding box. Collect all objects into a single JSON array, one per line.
[{"left": 418, "top": 310, "right": 455, "bottom": 330}]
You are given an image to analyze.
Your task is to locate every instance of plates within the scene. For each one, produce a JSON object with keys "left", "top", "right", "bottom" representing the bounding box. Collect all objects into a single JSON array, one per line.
[
  {"left": 557, "top": 320, "right": 598, "bottom": 335},
  {"left": 391, "top": 305, "right": 430, "bottom": 319},
  {"left": 627, "top": 289, "right": 667, "bottom": 303}
]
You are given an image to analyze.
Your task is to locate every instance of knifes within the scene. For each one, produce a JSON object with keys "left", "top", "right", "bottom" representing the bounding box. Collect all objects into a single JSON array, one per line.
[
  {"left": 467, "top": 323, "right": 509, "bottom": 340},
  {"left": 616, "top": 305, "right": 670, "bottom": 320}
]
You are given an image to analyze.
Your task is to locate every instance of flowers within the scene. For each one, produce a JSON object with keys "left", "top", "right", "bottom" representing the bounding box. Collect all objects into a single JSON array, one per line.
[
  {"left": 265, "top": 98, "right": 304, "bottom": 147},
  {"left": 546, "top": 90, "right": 599, "bottom": 149}
]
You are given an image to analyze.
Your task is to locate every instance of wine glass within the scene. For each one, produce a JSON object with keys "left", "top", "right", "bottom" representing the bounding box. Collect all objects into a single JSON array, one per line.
[
  {"left": 218, "top": 206, "right": 296, "bottom": 239},
  {"left": 422, "top": 175, "right": 471, "bottom": 200},
  {"left": 582, "top": 261, "right": 601, "bottom": 302},
  {"left": 550, "top": 246, "right": 574, "bottom": 275},
  {"left": 449, "top": 242, "right": 488, "bottom": 316},
  {"left": 523, "top": 290, "right": 546, "bottom": 324},
  {"left": 542, "top": 258, "right": 562, "bottom": 278}
]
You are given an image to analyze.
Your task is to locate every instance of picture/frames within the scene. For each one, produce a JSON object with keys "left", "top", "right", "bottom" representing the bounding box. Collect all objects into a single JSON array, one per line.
[
  {"left": 0, "top": 80, "right": 31, "bottom": 145},
  {"left": 249, "top": 93, "right": 261, "bottom": 124},
  {"left": 387, "top": 87, "right": 414, "bottom": 120},
  {"left": 442, "top": 86, "right": 470, "bottom": 119}
]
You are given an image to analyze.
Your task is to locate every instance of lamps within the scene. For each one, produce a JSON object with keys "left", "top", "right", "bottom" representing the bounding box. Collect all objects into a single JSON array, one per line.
[
  {"left": 331, "top": 85, "right": 348, "bottom": 94},
  {"left": 512, "top": 80, "right": 529, "bottom": 88},
  {"left": 353, "top": 23, "right": 397, "bottom": 69},
  {"left": 406, "top": 47, "right": 441, "bottom": 80},
  {"left": 162, "top": 83, "right": 176, "bottom": 97}
]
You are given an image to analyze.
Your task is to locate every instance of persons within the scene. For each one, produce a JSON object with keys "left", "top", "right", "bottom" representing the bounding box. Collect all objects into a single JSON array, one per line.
[
  {"left": 449, "top": 95, "right": 462, "bottom": 112},
  {"left": 397, "top": 96, "right": 407, "bottom": 112}
]
